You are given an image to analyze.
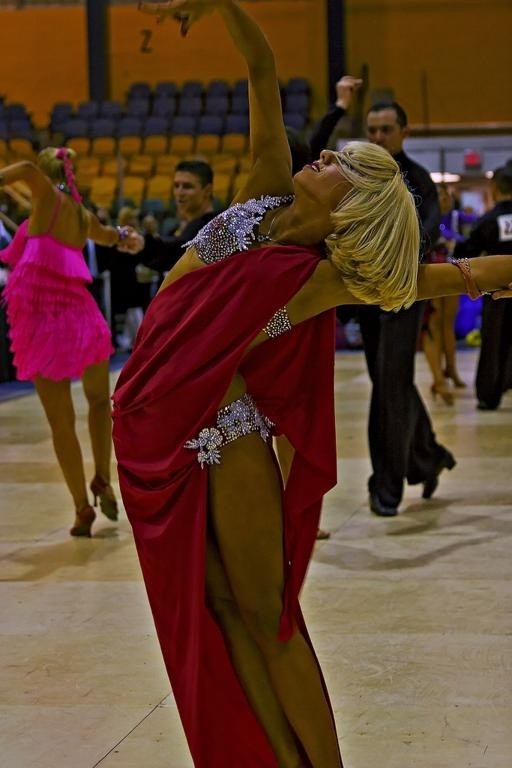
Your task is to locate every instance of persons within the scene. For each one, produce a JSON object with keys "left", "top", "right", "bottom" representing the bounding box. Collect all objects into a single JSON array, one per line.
[
  {"left": 111, "top": 1, "right": 512, "bottom": 766},
  {"left": 306, "top": 72, "right": 459, "bottom": 519},
  {"left": 0, "top": 144, "right": 133, "bottom": 537},
  {"left": 0, "top": 174, "right": 512, "bottom": 385},
  {"left": 410, "top": 158, "right": 511, "bottom": 417},
  {"left": 116, "top": 157, "right": 222, "bottom": 282}
]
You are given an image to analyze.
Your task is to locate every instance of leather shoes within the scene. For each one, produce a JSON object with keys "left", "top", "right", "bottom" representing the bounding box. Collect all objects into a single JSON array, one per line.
[
  {"left": 421, "top": 444, "right": 456, "bottom": 499},
  {"left": 367, "top": 473, "right": 397, "bottom": 517}
]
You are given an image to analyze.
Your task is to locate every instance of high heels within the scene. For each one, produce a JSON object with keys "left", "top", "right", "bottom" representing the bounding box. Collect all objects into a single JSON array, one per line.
[
  {"left": 427, "top": 368, "right": 500, "bottom": 411},
  {"left": 69, "top": 502, "right": 96, "bottom": 539},
  {"left": 90, "top": 467, "right": 120, "bottom": 522}
]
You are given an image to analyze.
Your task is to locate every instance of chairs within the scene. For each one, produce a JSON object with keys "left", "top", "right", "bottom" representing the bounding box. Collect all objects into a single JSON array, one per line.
[{"left": 2, "top": 73, "right": 316, "bottom": 235}]
